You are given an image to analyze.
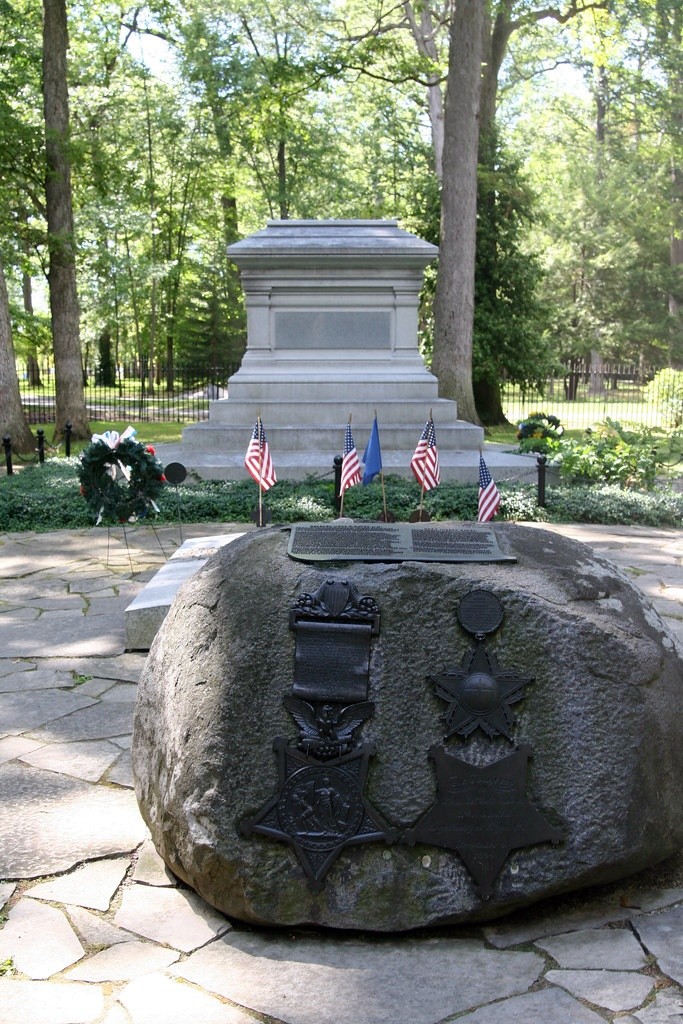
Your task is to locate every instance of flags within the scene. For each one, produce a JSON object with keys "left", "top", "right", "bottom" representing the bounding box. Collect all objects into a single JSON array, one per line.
[
  {"left": 338, "top": 421, "right": 364, "bottom": 497},
  {"left": 361, "top": 417, "right": 383, "bottom": 488},
  {"left": 410, "top": 418, "right": 441, "bottom": 492},
  {"left": 245, "top": 420, "right": 277, "bottom": 492},
  {"left": 478, "top": 456, "right": 501, "bottom": 522}
]
